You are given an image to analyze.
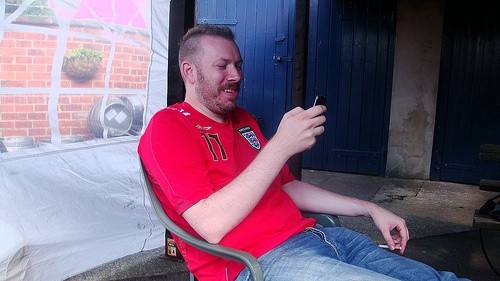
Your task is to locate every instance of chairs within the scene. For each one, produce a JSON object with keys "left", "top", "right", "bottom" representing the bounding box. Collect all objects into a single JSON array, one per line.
[{"left": 139, "top": 158, "right": 341, "bottom": 281}]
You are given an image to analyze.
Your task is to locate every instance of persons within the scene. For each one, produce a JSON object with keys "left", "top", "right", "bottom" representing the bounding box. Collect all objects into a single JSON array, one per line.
[{"left": 137, "top": 23, "right": 460, "bottom": 281}]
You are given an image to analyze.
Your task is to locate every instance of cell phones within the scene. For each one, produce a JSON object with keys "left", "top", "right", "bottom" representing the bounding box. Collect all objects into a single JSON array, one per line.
[{"left": 312, "top": 95, "right": 326, "bottom": 107}]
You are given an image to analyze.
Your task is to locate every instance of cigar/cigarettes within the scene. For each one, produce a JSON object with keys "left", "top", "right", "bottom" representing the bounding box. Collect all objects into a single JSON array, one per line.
[{"left": 378, "top": 244, "right": 402, "bottom": 249}]
